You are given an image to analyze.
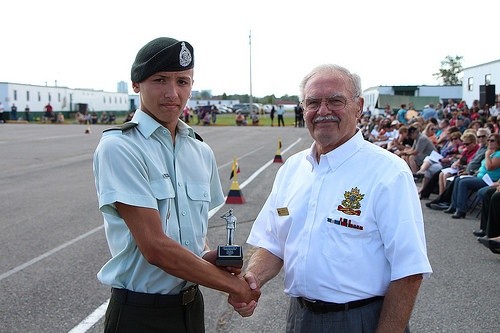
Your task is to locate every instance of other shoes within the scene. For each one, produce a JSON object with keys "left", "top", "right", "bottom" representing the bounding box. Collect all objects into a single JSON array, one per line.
[{"left": 414, "top": 174, "right": 500, "bottom": 255}]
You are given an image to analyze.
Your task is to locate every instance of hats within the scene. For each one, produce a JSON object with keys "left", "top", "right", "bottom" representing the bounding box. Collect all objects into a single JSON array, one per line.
[{"left": 131, "top": 37, "right": 195, "bottom": 83}]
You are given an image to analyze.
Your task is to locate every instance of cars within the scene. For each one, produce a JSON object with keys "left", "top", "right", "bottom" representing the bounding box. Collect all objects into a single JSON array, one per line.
[{"left": 196, "top": 101, "right": 280, "bottom": 114}]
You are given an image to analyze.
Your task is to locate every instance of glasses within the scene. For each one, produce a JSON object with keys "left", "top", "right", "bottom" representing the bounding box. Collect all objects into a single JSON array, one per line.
[
  {"left": 487, "top": 138, "right": 495, "bottom": 143},
  {"left": 430, "top": 128, "right": 436, "bottom": 130},
  {"left": 450, "top": 137, "right": 457, "bottom": 141},
  {"left": 462, "top": 141, "right": 473, "bottom": 146},
  {"left": 476, "top": 134, "right": 487, "bottom": 138},
  {"left": 300, "top": 96, "right": 361, "bottom": 112}
]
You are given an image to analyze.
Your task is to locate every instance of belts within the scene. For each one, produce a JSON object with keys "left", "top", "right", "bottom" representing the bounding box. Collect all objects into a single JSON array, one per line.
[
  {"left": 296, "top": 295, "right": 386, "bottom": 315},
  {"left": 110, "top": 286, "right": 198, "bottom": 305}
]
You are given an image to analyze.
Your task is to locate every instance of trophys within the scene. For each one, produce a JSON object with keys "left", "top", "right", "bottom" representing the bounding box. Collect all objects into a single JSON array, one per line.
[{"left": 214, "top": 208, "right": 245, "bottom": 268}]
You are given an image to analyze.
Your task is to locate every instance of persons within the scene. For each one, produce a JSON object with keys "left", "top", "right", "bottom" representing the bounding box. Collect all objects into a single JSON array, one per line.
[
  {"left": 355, "top": 97, "right": 500, "bottom": 256},
  {"left": 1, "top": 101, "right": 135, "bottom": 125},
  {"left": 179, "top": 100, "right": 307, "bottom": 129},
  {"left": 91, "top": 37, "right": 262, "bottom": 333},
  {"left": 226, "top": 64, "right": 435, "bottom": 333}
]
